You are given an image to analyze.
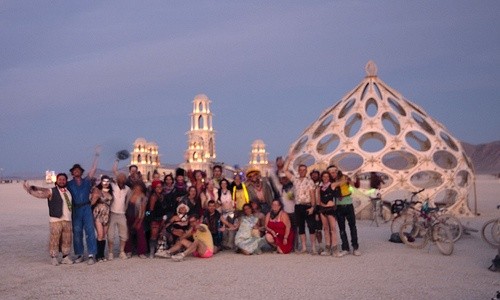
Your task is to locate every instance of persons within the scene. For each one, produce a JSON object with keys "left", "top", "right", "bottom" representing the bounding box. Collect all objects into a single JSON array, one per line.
[
  {"left": 24, "top": 145, "right": 360, "bottom": 265},
  {"left": 369, "top": 173, "right": 385, "bottom": 193}
]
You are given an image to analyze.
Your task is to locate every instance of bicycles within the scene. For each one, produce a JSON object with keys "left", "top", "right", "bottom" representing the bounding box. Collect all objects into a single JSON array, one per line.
[
  {"left": 481, "top": 203, "right": 500, "bottom": 248},
  {"left": 389, "top": 187, "right": 478, "bottom": 256},
  {"left": 364, "top": 175, "right": 391, "bottom": 228}
]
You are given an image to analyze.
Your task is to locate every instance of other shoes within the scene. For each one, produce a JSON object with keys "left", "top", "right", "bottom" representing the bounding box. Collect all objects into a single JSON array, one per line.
[
  {"left": 235, "top": 247, "right": 239, "bottom": 253},
  {"left": 88, "top": 257, "right": 95, "bottom": 265},
  {"left": 138, "top": 254, "right": 147, "bottom": 259},
  {"left": 107, "top": 253, "right": 114, "bottom": 260},
  {"left": 74, "top": 254, "right": 84, "bottom": 263},
  {"left": 60, "top": 255, "right": 70, "bottom": 264},
  {"left": 155, "top": 250, "right": 172, "bottom": 258},
  {"left": 172, "top": 252, "right": 185, "bottom": 261},
  {"left": 96, "top": 256, "right": 107, "bottom": 263},
  {"left": 50, "top": 257, "right": 59, "bottom": 265},
  {"left": 293, "top": 246, "right": 361, "bottom": 257},
  {"left": 119, "top": 252, "right": 128, "bottom": 259},
  {"left": 127, "top": 252, "right": 132, "bottom": 257}
]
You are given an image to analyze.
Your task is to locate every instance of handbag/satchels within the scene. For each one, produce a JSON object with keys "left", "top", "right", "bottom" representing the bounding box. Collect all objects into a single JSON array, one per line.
[{"left": 388, "top": 232, "right": 416, "bottom": 243}]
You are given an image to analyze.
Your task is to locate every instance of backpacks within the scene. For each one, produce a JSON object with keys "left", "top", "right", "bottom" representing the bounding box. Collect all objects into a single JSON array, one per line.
[{"left": 488, "top": 254, "right": 500, "bottom": 272}]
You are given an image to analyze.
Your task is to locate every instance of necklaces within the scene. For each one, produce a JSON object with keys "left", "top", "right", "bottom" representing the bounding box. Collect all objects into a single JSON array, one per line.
[{"left": 222, "top": 190, "right": 227, "bottom": 195}]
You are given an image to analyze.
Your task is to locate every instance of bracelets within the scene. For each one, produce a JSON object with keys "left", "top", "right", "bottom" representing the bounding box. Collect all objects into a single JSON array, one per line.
[{"left": 96, "top": 153, "right": 100, "bottom": 156}]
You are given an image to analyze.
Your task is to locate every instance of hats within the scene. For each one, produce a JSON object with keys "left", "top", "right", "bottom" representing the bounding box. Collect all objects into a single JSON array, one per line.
[
  {"left": 249, "top": 198, "right": 262, "bottom": 209},
  {"left": 69, "top": 164, "right": 84, "bottom": 173},
  {"left": 176, "top": 203, "right": 190, "bottom": 214},
  {"left": 151, "top": 180, "right": 162, "bottom": 189},
  {"left": 246, "top": 166, "right": 261, "bottom": 178},
  {"left": 176, "top": 168, "right": 185, "bottom": 176}
]
